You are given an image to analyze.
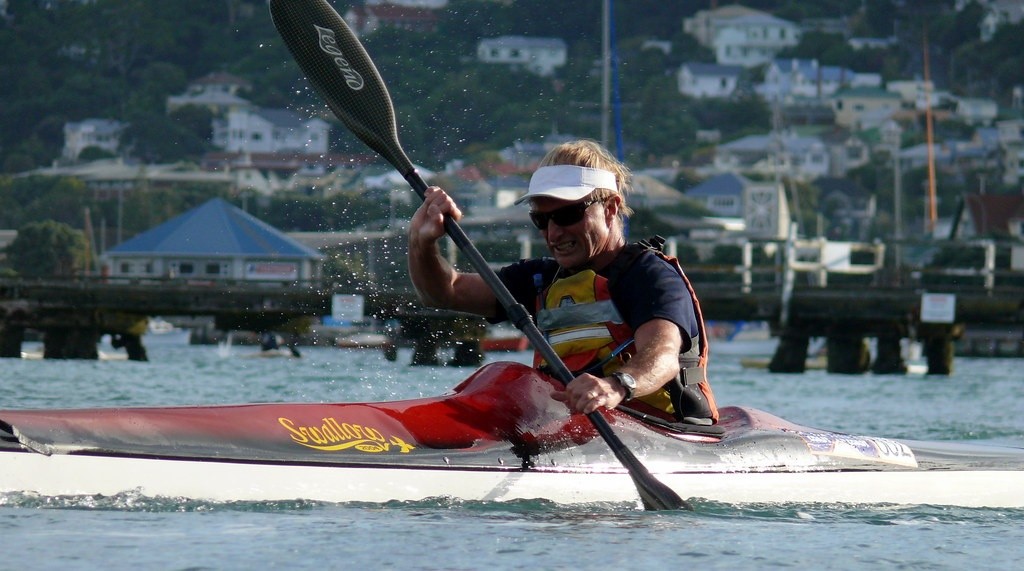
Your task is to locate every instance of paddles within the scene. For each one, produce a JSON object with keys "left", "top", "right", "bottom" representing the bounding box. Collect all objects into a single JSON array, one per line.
[{"left": 270, "top": 0, "right": 697, "bottom": 511}]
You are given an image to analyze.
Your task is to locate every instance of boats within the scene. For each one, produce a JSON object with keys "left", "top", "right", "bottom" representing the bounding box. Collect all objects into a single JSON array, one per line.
[{"left": 0, "top": 359, "right": 1024, "bottom": 510}]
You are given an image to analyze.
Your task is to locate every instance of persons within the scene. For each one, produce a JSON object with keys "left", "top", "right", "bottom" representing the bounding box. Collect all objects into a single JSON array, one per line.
[{"left": 408, "top": 138, "right": 719, "bottom": 424}]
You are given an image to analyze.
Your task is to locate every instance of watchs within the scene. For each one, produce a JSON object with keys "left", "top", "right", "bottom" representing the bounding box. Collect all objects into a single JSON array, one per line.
[{"left": 611, "top": 372, "right": 636, "bottom": 402}]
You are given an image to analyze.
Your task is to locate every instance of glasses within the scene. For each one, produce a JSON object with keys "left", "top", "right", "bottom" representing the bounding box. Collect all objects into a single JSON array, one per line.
[{"left": 528, "top": 194, "right": 605, "bottom": 230}]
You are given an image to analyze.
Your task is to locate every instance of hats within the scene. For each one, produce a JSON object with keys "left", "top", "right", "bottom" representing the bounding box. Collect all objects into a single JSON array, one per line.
[{"left": 513, "top": 165, "right": 617, "bottom": 205}]
